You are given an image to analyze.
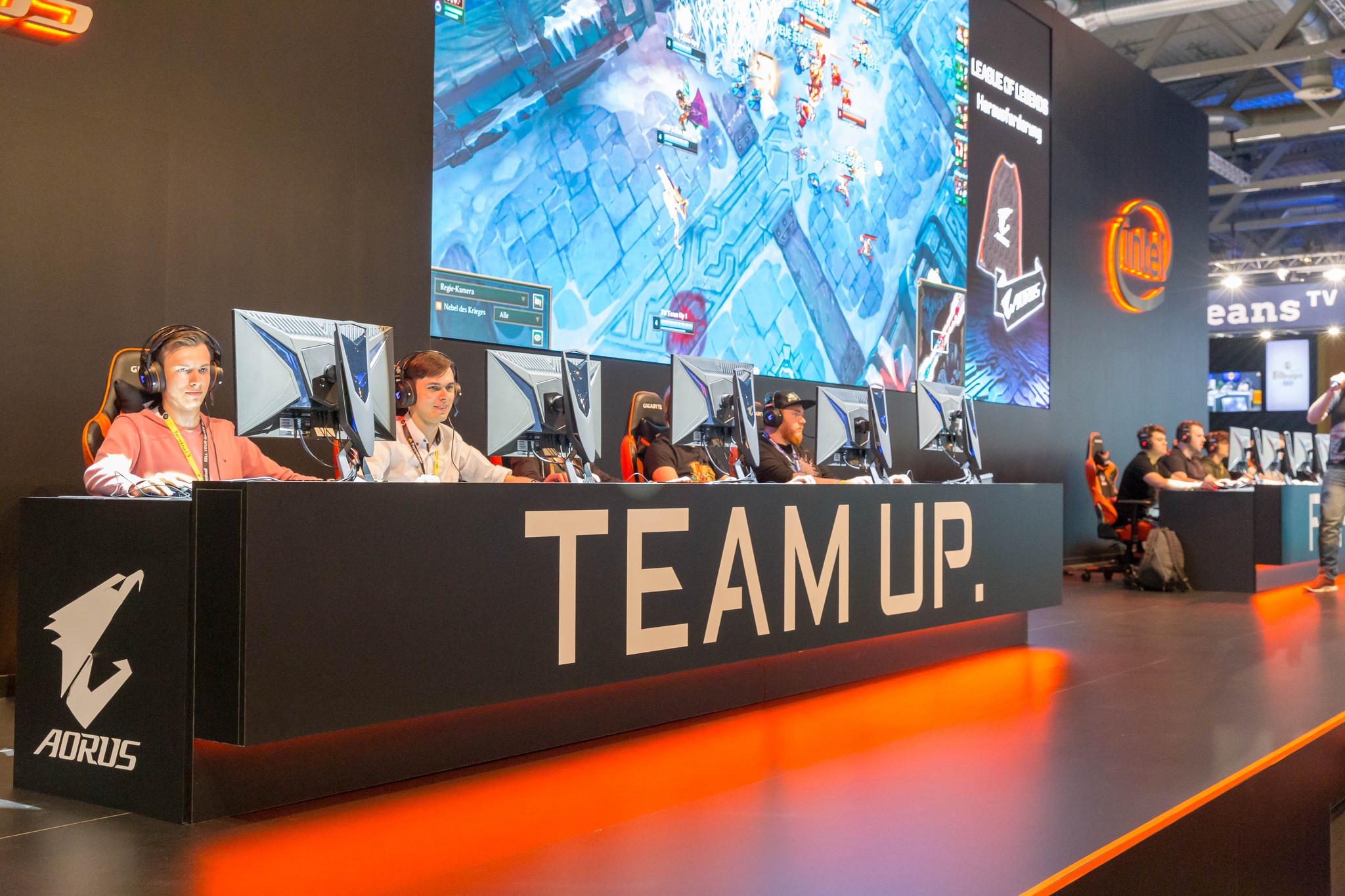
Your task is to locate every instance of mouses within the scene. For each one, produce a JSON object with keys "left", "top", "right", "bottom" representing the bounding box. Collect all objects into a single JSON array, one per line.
[{"left": 149, "top": 483, "right": 192, "bottom": 498}]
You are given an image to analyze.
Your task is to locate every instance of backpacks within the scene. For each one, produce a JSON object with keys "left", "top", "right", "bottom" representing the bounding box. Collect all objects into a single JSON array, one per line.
[{"left": 1141, "top": 527, "right": 1185, "bottom": 590}]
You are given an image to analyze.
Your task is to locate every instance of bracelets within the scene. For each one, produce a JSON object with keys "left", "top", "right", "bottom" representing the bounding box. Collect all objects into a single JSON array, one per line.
[{"left": 837, "top": 478, "right": 845, "bottom": 485}]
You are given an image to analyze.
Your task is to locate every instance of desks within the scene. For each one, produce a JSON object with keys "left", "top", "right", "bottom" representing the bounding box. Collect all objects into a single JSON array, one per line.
[
  {"left": 1158, "top": 484, "right": 1345, "bottom": 594},
  {"left": 12, "top": 481, "right": 1063, "bottom": 825}
]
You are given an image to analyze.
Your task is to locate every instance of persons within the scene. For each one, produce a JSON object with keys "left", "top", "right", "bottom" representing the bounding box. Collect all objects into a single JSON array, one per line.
[
  {"left": 350, "top": 352, "right": 546, "bottom": 484},
  {"left": 1201, "top": 430, "right": 1255, "bottom": 486},
  {"left": 84, "top": 325, "right": 339, "bottom": 500},
  {"left": 1301, "top": 369, "right": 1345, "bottom": 594},
  {"left": 1111, "top": 424, "right": 1218, "bottom": 553},
  {"left": 510, "top": 453, "right": 629, "bottom": 484},
  {"left": 1160, "top": 421, "right": 1236, "bottom": 488},
  {"left": 644, "top": 385, "right": 737, "bottom": 482},
  {"left": 742, "top": 390, "right": 912, "bottom": 485}
]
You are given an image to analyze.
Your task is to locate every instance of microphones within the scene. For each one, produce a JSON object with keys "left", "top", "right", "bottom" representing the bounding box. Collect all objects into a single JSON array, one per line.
[
  {"left": 452, "top": 405, "right": 459, "bottom": 417},
  {"left": 209, "top": 391, "right": 214, "bottom": 406},
  {"left": 1330, "top": 381, "right": 1339, "bottom": 387}
]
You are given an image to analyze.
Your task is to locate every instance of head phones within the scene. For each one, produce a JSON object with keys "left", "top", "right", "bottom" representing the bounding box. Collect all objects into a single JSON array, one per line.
[
  {"left": 1207, "top": 432, "right": 1219, "bottom": 452},
  {"left": 395, "top": 350, "right": 462, "bottom": 409},
  {"left": 1178, "top": 420, "right": 1192, "bottom": 443},
  {"left": 762, "top": 390, "right": 783, "bottom": 427},
  {"left": 1140, "top": 423, "right": 1153, "bottom": 450},
  {"left": 138, "top": 325, "right": 223, "bottom": 394}
]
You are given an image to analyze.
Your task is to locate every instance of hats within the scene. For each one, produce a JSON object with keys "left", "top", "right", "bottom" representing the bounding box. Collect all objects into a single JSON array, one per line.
[{"left": 764, "top": 390, "right": 816, "bottom": 414}]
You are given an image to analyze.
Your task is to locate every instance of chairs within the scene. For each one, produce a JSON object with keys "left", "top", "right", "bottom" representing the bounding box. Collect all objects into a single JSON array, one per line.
[
  {"left": 81, "top": 348, "right": 150, "bottom": 468},
  {"left": 1082, "top": 431, "right": 1151, "bottom": 581},
  {"left": 621, "top": 391, "right": 669, "bottom": 482}
]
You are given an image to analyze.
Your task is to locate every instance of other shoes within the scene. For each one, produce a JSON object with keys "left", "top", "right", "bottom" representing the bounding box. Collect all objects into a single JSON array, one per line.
[{"left": 1302, "top": 574, "right": 1336, "bottom": 592}]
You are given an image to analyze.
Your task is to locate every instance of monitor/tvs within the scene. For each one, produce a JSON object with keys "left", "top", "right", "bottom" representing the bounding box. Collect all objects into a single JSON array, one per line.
[
  {"left": 914, "top": 380, "right": 982, "bottom": 484},
  {"left": 231, "top": 309, "right": 397, "bottom": 482},
  {"left": 814, "top": 384, "right": 893, "bottom": 484},
  {"left": 1222, "top": 371, "right": 1241, "bottom": 382},
  {"left": 484, "top": 349, "right": 602, "bottom": 483},
  {"left": 1226, "top": 426, "right": 1330, "bottom": 489},
  {"left": 669, "top": 353, "right": 760, "bottom": 484}
]
